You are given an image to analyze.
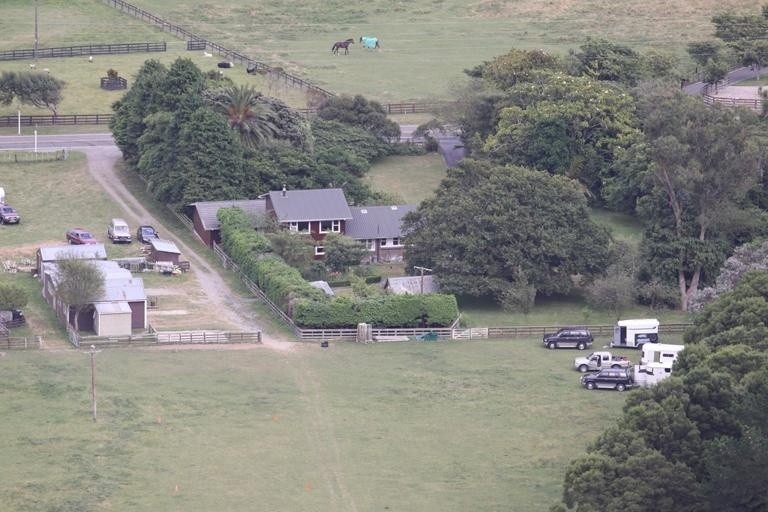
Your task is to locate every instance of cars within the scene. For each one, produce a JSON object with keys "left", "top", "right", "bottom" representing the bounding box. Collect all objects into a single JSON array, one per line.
[
  {"left": 135, "top": 225, "right": 160, "bottom": 246},
  {"left": 66, "top": 228, "right": 98, "bottom": 249}
]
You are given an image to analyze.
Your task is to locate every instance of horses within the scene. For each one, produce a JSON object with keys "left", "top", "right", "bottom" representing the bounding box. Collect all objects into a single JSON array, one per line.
[
  {"left": 331, "top": 38, "right": 355, "bottom": 56},
  {"left": 359, "top": 36, "right": 380, "bottom": 49}
]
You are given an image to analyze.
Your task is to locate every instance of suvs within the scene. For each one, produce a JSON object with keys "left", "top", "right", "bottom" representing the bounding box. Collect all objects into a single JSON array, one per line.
[
  {"left": 541, "top": 325, "right": 595, "bottom": 352},
  {"left": 106, "top": 218, "right": 134, "bottom": 245},
  {"left": 0, "top": 204, "right": 21, "bottom": 226},
  {"left": 579, "top": 367, "right": 634, "bottom": 392}
]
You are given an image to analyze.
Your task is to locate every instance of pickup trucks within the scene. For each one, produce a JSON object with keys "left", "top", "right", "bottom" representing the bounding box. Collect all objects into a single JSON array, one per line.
[{"left": 575, "top": 350, "right": 632, "bottom": 371}]
